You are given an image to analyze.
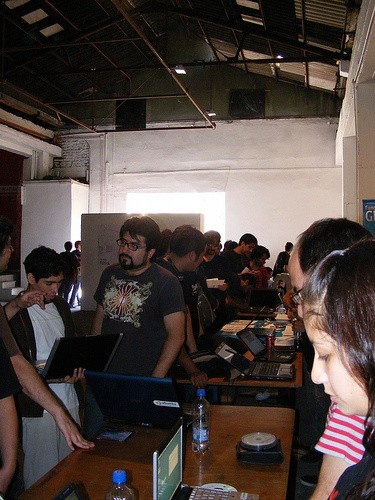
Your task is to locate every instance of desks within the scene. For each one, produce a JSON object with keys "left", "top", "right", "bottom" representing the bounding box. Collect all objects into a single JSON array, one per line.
[
  {"left": 17, "top": 405, "right": 296, "bottom": 500},
  {"left": 238, "top": 308, "right": 276, "bottom": 319},
  {"left": 182, "top": 320, "right": 305, "bottom": 403}
]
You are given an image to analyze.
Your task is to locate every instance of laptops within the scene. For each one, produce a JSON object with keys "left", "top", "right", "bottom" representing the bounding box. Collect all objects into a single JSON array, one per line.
[
  {"left": 84, "top": 369, "right": 207, "bottom": 427},
  {"left": 217, "top": 304, "right": 266, "bottom": 337},
  {"left": 236, "top": 326, "right": 295, "bottom": 362},
  {"left": 213, "top": 340, "right": 296, "bottom": 381},
  {"left": 152, "top": 415, "right": 259, "bottom": 500},
  {"left": 247, "top": 288, "right": 279, "bottom": 310},
  {"left": 30, "top": 331, "right": 123, "bottom": 385}
]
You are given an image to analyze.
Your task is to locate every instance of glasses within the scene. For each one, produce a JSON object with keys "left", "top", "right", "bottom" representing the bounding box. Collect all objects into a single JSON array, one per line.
[
  {"left": 290, "top": 288, "right": 305, "bottom": 306},
  {"left": 116, "top": 238, "right": 147, "bottom": 251}
]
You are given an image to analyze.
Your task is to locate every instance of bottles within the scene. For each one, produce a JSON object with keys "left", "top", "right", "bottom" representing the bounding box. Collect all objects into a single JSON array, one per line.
[
  {"left": 105, "top": 469, "right": 134, "bottom": 500},
  {"left": 265, "top": 325, "right": 276, "bottom": 350},
  {"left": 192, "top": 389, "right": 210, "bottom": 453}
]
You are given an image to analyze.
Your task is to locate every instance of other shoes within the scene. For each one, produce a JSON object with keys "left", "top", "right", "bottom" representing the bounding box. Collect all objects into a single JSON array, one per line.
[{"left": 300, "top": 475, "right": 319, "bottom": 487}]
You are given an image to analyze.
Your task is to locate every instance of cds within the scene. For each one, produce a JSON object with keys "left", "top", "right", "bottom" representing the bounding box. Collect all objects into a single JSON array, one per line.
[{"left": 240, "top": 431, "right": 277, "bottom": 449}]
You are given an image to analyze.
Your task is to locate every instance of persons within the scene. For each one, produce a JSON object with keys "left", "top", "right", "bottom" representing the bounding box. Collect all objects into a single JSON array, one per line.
[
  {"left": 0, "top": 340, "right": 20, "bottom": 500},
  {"left": 274, "top": 217, "right": 375, "bottom": 500},
  {"left": 56, "top": 241, "right": 82, "bottom": 307},
  {"left": 87, "top": 217, "right": 270, "bottom": 381},
  {"left": 1, "top": 215, "right": 97, "bottom": 451},
  {"left": 9, "top": 245, "right": 83, "bottom": 489}
]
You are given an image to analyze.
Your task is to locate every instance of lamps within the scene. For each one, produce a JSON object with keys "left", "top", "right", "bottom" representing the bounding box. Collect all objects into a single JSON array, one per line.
[
  {"left": 174, "top": 64, "right": 186, "bottom": 74},
  {"left": 204, "top": 109, "right": 216, "bottom": 116}
]
